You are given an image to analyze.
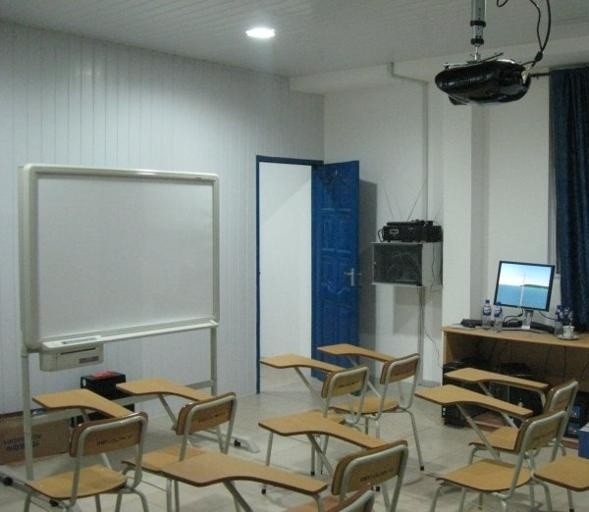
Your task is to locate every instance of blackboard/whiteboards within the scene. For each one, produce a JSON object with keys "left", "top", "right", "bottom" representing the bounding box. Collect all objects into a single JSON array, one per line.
[{"left": 15, "top": 162, "right": 220, "bottom": 351}]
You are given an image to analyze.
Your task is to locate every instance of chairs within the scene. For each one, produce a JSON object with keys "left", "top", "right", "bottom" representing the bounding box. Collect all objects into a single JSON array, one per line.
[
  {"left": 259, "top": 366, "right": 381, "bottom": 495},
  {"left": 285, "top": 439, "right": 409, "bottom": 512},
  {"left": 429, "top": 410, "right": 569, "bottom": 512},
  {"left": 328, "top": 482, "right": 375, "bottom": 512},
  {"left": 468, "top": 379, "right": 578, "bottom": 467},
  {"left": 115, "top": 392, "right": 237, "bottom": 512},
  {"left": 23, "top": 410, "right": 150, "bottom": 512},
  {"left": 320, "top": 353, "right": 424, "bottom": 473}
]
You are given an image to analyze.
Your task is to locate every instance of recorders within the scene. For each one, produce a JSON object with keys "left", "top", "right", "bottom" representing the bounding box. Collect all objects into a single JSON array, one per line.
[{"left": 382, "top": 218, "right": 442, "bottom": 243}]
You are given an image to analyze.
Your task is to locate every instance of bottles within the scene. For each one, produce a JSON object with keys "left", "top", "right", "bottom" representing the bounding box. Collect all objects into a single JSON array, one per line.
[
  {"left": 482, "top": 299, "right": 492, "bottom": 329},
  {"left": 494, "top": 301, "right": 503, "bottom": 332}
]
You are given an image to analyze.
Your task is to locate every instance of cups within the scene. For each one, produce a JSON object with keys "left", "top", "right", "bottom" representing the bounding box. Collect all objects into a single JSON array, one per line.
[{"left": 563, "top": 326, "right": 574, "bottom": 336}]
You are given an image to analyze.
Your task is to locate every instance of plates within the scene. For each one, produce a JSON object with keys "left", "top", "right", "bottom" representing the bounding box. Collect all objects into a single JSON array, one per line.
[{"left": 558, "top": 335, "right": 579, "bottom": 339}]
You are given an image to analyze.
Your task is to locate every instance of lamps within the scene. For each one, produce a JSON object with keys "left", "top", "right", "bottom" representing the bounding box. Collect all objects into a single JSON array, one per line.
[{"left": 245, "top": 26, "right": 276, "bottom": 40}]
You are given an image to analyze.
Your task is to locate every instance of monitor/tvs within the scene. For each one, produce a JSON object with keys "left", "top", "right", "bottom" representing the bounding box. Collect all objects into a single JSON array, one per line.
[{"left": 494, "top": 260, "right": 555, "bottom": 334}]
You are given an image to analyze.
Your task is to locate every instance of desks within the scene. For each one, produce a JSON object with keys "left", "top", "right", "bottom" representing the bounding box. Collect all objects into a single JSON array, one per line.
[
  {"left": 532, "top": 455, "right": 589, "bottom": 491},
  {"left": 316, "top": 343, "right": 396, "bottom": 401},
  {"left": 444, "top": 367, "right": 548, "bottom": 429},
  {"left": 414, "top": 384, "right": 533, "bottom": 460},
  {"left": 259, "top": 352, "right": 346, "bottom": 404},
  {"left": 32, "top": 387, "right": 135, "bottom": 470},
  {"left": 441, "top": 323, "right": 589, "bottom": 399},
  {"left": 258, "top": 413, "right": 389, "bottom": 479},
  {"left": 116, "top": 377, "right": 215, "bottom": 448},
  {"left": 161, "top": 451, "right": 329, "bottom": 512}
]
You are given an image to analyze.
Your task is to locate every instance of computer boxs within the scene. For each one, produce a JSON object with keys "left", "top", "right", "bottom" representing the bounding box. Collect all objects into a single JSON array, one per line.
[{"left": 440, "top": 361, "right": 490, "bottom": 421}]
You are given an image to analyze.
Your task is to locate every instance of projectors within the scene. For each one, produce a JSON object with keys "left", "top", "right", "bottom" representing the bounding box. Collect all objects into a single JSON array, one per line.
[{"left": 434, "top": 57, "right": 533, "bottom": 106}]
